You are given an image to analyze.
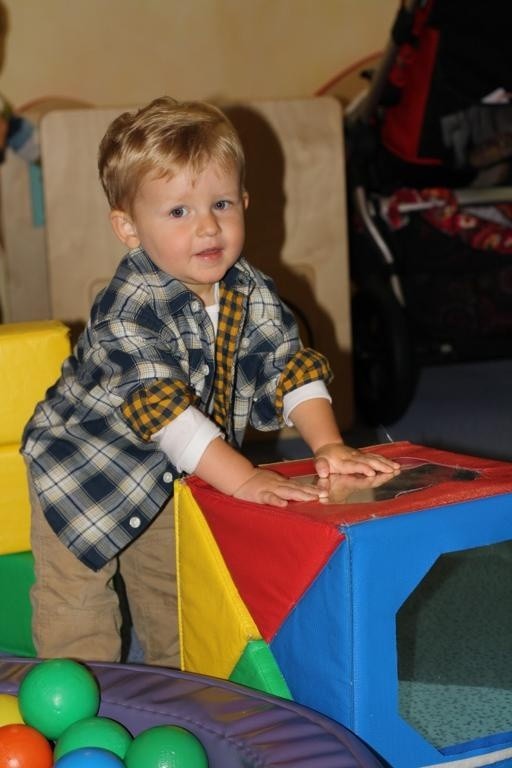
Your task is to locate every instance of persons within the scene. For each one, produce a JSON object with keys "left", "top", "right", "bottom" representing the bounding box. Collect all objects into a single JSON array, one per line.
[{"left": 16, "top": 93, "right": 403, "bottom": 669}]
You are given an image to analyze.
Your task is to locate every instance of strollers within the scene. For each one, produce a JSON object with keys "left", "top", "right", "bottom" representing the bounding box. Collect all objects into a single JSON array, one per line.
[{"left": 344, "top": 0, "right": 511, "bottom": 434}]
